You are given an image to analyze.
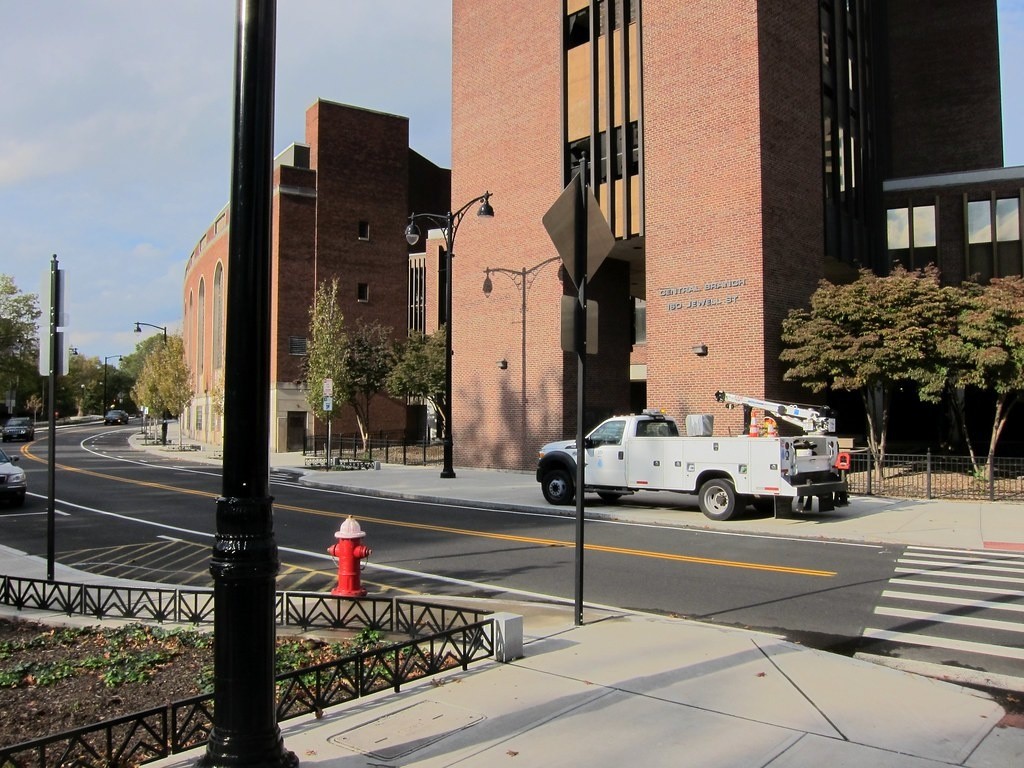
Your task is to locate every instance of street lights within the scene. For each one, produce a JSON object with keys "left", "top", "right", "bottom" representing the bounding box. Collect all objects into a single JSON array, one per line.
[
  {"left": 132, "top": 321, "right": 170, "bottom": 444},
  {"left": 101, "top": 354, "right": 123, "bottom": 422},
  {"left": 403, "top": 190, "right": 496, "bottom": 477}
]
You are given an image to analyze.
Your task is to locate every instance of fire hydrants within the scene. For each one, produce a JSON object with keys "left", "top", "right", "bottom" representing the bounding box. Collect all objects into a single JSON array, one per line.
[{"left": 326, "top": 514, "right": 374, "bottom": 595}]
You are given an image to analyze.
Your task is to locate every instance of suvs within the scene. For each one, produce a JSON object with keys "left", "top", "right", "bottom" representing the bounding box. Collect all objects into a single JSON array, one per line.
[{"left": 2, "top": 417, "right": 35, "bottom": 443}]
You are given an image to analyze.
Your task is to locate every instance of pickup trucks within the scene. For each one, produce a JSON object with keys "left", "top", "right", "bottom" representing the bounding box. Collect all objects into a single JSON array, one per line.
[{"left": 533, "top": 388, "right": 849, "bottom": 522}]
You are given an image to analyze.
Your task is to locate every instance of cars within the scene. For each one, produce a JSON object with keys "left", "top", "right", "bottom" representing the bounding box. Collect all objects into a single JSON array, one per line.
[
  {"left": 103, "top": 409, "right": 130, "bottom": 425},
  {"left": 0, "top": 448, "right": 27, "bottom": 509}
]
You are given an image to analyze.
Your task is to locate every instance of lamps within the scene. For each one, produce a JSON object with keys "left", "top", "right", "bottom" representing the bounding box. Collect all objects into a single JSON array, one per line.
[
  {"left": 496, "top": 359, "right": 507, "bottom": 369},
  {"left": 691, "top": 344, "right": 709, "bottom": 356}
]
